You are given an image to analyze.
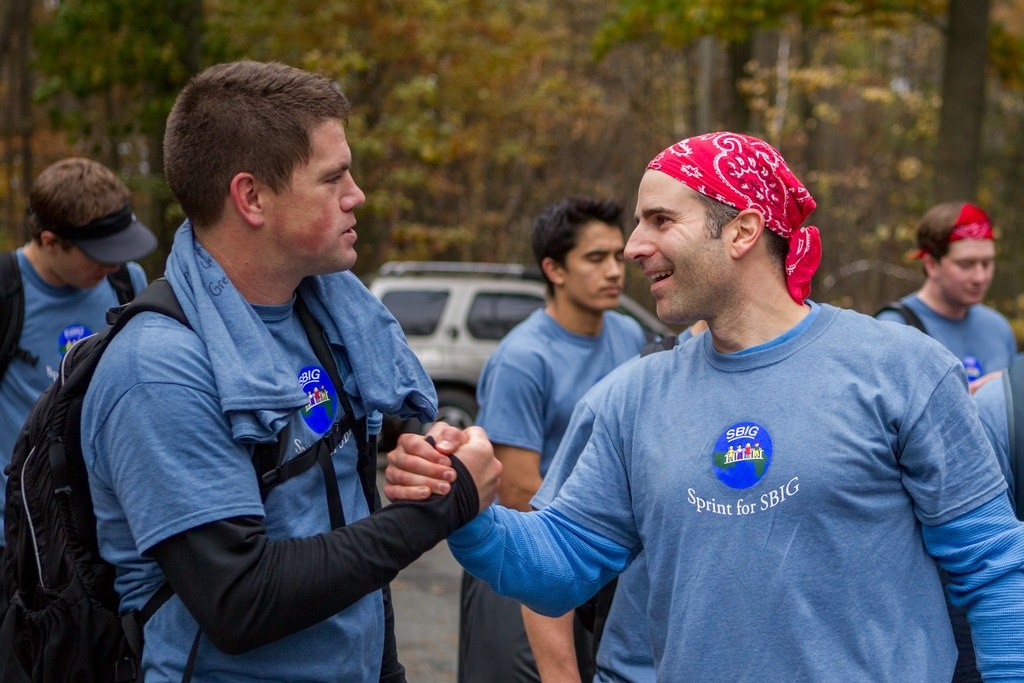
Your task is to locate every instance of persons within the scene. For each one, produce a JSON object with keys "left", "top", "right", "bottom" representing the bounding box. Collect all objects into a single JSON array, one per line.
[
  {"left": 474, "top": 191, "right": 715, "bottom": 683},
  {"left": 77, "top": 55, "right": 506, "bottom": 682},
  {"left": 869, "top": 197, "right": 1017, "bottom": 395},
  {"left": 383, "top": 128, "right": 1023, "bottom": 682},
  {"left": 0, "top": 155, "right": 151, "bottom": 611}
]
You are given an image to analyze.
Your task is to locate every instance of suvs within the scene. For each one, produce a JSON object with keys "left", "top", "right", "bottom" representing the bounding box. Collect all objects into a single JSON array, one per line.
[{"left": 368, "top": 260, "right": 680, "bottom": 437}]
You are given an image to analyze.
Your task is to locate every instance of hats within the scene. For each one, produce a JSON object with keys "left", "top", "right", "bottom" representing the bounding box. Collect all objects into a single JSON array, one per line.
[{"left": 24, "top": 188, "right": 158, "bottom": 265}]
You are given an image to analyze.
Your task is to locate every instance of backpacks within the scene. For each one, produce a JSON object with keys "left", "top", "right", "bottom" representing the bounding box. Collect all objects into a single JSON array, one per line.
[{"left": 1, "top": 274, "right": 277, "bottom": 683}]
